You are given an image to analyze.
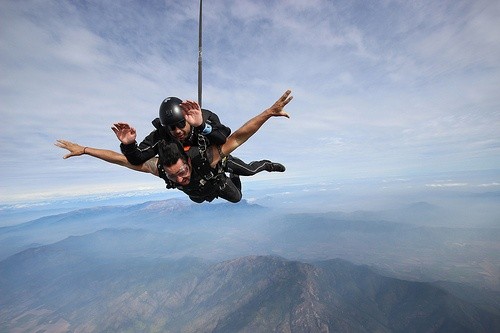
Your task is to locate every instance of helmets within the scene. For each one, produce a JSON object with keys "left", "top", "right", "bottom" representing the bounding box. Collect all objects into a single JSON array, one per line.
[{"left": 159, "top": 97, "right": 185, "bottom": 126}]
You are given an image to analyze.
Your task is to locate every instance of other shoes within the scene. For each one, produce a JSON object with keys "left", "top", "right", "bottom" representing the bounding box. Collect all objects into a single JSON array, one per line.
[
  {"left": 272, "top": 163, "right": 286, "bottom": 172},
  {"left": 230, "top": 173, "right": 239, "bottom": 178}
]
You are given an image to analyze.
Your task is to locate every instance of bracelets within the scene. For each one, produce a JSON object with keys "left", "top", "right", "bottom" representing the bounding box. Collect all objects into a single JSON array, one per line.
[{"left": 84, "top": 147, "right": 88, "bottom": 154}]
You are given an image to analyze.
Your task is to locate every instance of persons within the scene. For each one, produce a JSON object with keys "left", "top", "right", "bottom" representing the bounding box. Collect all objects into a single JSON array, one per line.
[
  {"left": 111, "top": 97, "right": 286, "bottom": 177},
  {"left": 52, "top": 88, "right": 294, "bottom": 203}
]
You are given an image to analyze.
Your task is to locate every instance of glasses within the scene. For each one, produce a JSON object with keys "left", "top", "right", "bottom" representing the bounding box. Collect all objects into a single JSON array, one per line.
[
  {"left": 168, "top": 119, "right": 186, "bottom": 131},
  {"left": 166, "top": 164, "right": 189, "bottom": 182}
]
